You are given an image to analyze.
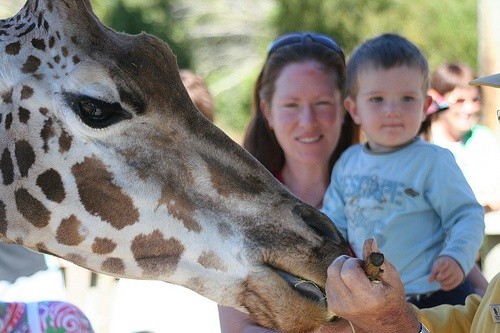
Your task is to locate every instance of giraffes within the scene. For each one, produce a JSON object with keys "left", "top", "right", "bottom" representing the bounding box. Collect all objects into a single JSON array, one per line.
[{"left": 0, "top": 0, "right": 356, "bottom": 331}]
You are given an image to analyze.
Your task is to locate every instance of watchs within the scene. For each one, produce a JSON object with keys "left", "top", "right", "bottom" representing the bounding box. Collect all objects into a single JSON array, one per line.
[{"left": 419, "top": 322, "right": 429, "bottom": 333}]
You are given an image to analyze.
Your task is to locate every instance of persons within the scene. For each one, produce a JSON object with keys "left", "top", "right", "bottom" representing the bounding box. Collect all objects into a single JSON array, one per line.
[
  {"left": 217, "top": 32, "right": 489, "bottom": 332},
  {"left": 179, "top": 68, "right": 214, "bottom": 123},
  {"left": 423, "top": 60, "right": 500, "bottom": 278},
  {"left": 320, "top": 32, "right": 485, "bottom": 308},
  {"left": 313, "top": 236, "right": 500, "bottom": 332}
]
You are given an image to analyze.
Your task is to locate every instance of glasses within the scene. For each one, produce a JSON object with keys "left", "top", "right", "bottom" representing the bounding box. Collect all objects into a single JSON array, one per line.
[{"left": 257, "top": 32, "right": 346, "bottom": 81}]
